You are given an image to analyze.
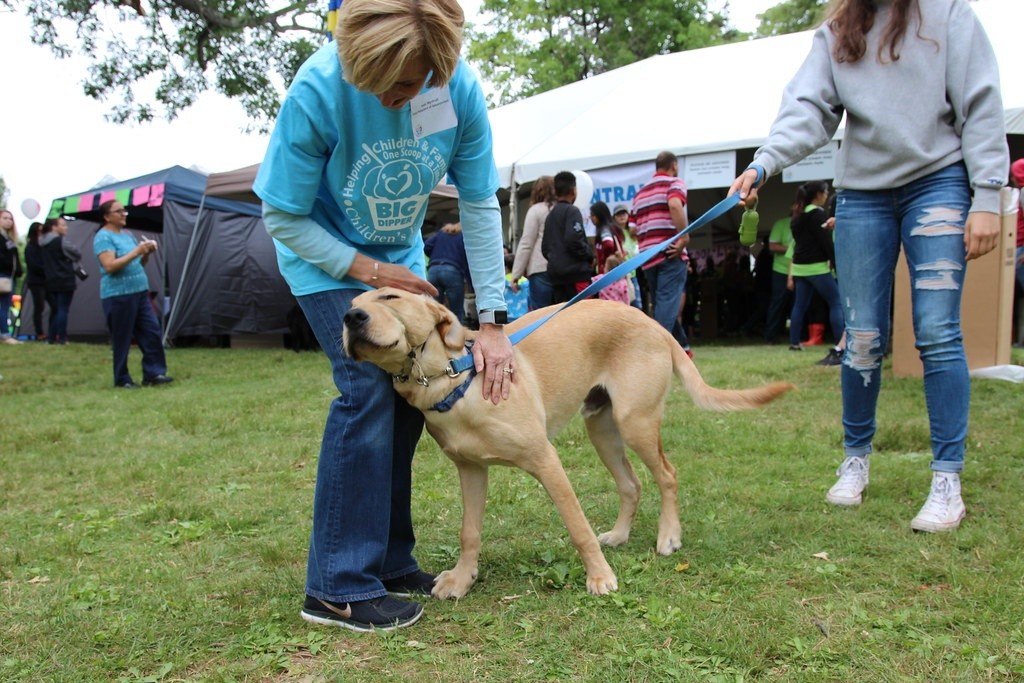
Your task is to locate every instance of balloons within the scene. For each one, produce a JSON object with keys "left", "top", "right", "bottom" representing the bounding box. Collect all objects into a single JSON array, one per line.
[{"left": 21, "top": 199, "right": 40, "bottom": 219}]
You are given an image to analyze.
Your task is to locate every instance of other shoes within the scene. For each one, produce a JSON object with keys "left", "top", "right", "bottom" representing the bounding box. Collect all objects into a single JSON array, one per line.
[
  {"left": 815, "top": 349, "right": 846, "bottom": 367},
  {"left": 116, "top": 381, "right": 136, "bottom": 389},
  {"left": 141, "top": 374, "right": 174, "bottom": 386},
  {"left": 684, "top": 350, "right": 694, "bottom": 358},
  {"left": 0, "top": 336, "right": 22, "bottom": 345}
]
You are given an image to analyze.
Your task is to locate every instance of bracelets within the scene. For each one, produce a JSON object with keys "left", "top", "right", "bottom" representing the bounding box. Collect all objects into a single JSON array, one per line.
[{"left": 373, "top": 263, "right": 379, "bottom": 280}]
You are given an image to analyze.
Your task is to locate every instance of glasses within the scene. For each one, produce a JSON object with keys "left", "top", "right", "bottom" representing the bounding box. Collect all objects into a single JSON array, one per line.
[{"left": 107, "top": 208, "right": 126, "bottom": 215}]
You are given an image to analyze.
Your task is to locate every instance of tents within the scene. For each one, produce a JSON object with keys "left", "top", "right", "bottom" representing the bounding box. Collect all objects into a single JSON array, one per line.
[
  {"left": 162, "top": 163, "right": 459, "bottom": 344},
  {"left": 16, "top": 165, "right": 207, "bottom": 347},
  {"left": 487, "top": 0, "right": 1024, "bottom": 187}
]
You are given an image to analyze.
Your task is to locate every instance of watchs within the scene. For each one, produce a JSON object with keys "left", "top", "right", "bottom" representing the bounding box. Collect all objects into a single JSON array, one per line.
[{"left": 479, "top": 310, "right": 507, "bottom": 325}]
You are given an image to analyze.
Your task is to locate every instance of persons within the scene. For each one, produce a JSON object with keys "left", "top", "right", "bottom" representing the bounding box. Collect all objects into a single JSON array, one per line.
[
  {"left": 727, "top": 0, "right": 1010, "bottom": 533},
  {"left": 1012, "top": 158, "right": 1024, "bottom": 349},
  {"left": 93, "top": 200, "right": 173, "bottom": 388},
  {"left": 0, "top": 209, "right": 81, "bottom": 344},
  {"left": 424, "top": 223, "right": 529, "bottom": 326},
  {"left": 251, "top": 0, "right": 519, "bottom": 631},
  {"left": 765, "top": 181, "right": 848, "bottom": 365},
  {"left": 503, "top": 152, "right": 690, "bottom": 358}
]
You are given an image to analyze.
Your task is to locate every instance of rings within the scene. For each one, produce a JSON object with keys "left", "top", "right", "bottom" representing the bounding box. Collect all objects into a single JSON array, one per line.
[{"left": 504, "top": 368, "right": 513, "bottom": 373}]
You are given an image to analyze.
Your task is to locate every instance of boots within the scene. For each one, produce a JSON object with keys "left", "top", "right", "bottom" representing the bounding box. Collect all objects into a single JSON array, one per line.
[{"left": 800, "top": 323, "right": 825, "bottom": 346}]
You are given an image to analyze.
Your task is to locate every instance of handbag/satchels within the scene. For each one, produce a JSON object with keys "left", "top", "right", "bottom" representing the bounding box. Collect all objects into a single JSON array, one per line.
[
  {"left": 591, "top": 272, "right": 630, "bottom": 306},
  {"left": 0, "top": 277, "right": 13, "bottom": 292}
]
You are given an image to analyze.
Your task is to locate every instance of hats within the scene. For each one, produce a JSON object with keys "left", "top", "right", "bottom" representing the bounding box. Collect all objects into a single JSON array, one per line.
[{"left": 613, "top": 205, "right": 630, "bottom": 219}]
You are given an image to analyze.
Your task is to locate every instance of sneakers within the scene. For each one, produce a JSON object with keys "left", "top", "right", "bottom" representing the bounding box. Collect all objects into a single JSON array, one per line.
[
  {"left": 827, "top": 455, "right": 868, "bottom": 506},
  {"left": 911, "top": 472, "right": 966, "bottom": 533},
  {"left": 300, "top": 593, "right": 425, "bottom": 634},
  {"left": 380, "top": 568, "right": 436, "bottom": 600}
]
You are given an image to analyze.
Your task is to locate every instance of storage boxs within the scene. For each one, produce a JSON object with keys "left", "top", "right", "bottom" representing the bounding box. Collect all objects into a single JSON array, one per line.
[{"left": 892, "top": 186, "right": 1018, "bottom": 381}]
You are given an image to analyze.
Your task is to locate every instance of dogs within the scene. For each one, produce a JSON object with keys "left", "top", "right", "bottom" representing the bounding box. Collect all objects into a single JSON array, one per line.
[{"left": 341, "top": 285, "right": 803, "bottom": 602}]
